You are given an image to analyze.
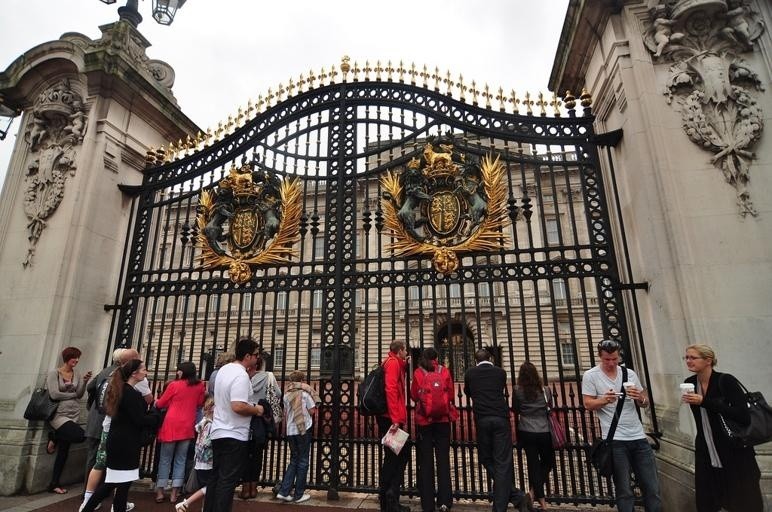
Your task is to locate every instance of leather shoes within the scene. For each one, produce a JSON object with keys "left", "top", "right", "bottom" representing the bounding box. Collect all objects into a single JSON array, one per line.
[
  {"left": 515, "top": 495, "right": 528, "bottom": 511},
  {"left": 382, "top": 504, "right": 410, "bottom": 512}
]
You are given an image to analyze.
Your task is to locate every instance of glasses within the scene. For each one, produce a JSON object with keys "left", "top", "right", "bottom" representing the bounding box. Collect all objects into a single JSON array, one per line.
[
  {"left": 682, "top": 355, "right": 704, "bottom": 361},
  {"left": 597, "top": 340, "right": 620, "bottom": 348}
]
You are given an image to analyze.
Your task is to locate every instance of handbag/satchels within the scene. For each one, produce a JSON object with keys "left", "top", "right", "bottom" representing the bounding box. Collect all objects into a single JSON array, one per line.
[
  {"left": 96, "top": 376, "right": 115, "bottom": 414},
  {"left": 140, "top": 399, "right": 163, "bottom": 447},
  {"left": 184, "top": 468, "right": 200, "bottom": 494},
  {"left": 590, "top": 436, "right": 613, "bottom": 478},
  {"left": 24, "top": 388, "right": 60, "bottom": 421},
  {"left": 717, "top": 391, "right": 772, "bottom": 450},
  {"left": 546, "top": 406, "right": 567, "bottom": 449}
]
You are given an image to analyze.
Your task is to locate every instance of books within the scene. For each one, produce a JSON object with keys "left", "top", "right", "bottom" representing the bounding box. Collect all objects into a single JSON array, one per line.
[{"left": 381, "top": 425, "right": 410, "bottom": 457}]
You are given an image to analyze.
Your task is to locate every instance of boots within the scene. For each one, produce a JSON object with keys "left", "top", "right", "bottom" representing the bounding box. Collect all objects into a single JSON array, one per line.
[
  {"left": 251, "top": 482, "right": 257, "bottom": 498},
  {"left": 238, "top": 482, "right": 251, "bottom": 499}
]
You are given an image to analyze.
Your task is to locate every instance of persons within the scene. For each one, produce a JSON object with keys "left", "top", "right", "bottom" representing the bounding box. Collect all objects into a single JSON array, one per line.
[
  {"left": 374, "top": 339, "right": 412, "bottom": 511},
  {"left": 464, "top": 349, "right": 529, "bottom": 511},
  {"left": 275, "top": 371, "right": 314, "bottom": 502},
  {"left": 48, "top": 347, "right": 92, "bottom": 494},
  {"left": 409, "top": 347, "right": 455, "bottom": 511},
  {"left": 153, "top": 338, "right": 283, "bottom": 512},
  {"left": 79, "top": 348, "right": 154, "bottom": 512},
  {"left": 681, "top": 344, "right": 764, "bottom": 511},
  {"left": 511, "top": 361, "right": 554, "bottom": 512},
  {"left": 581, "top": 338, "right": 665, "bottom": 512}
]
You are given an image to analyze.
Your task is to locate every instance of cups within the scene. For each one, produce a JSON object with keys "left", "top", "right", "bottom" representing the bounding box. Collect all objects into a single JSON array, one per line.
[
  {"left": 622, "top": 381, "right": 636, "bottom": 397},
  {"left": 680, "top": 382, "right": 696, "bottom": 396}
]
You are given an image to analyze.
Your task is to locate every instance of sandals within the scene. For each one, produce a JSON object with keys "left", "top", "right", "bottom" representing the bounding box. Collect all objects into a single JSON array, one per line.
[
  {"left": 46, "top": 431, "right": 56, "bottom": 455},
  {"left": 175, "top": 498, "right": 189, "bottom": 512},
  {"left": 46, "top": 485, "right": 68, "bottom": 494}
]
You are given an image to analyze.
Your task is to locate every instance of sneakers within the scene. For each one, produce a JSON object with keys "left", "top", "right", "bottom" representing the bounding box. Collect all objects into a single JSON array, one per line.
[
  {"left": 111, "top": 500, "right": 135, "bottom": 512},
  {"left": 294, "top": 494, "right": 310, "bottom": 503},
  {"left": 276, "top": 493, "right": 293, "bottom": 501},
  {"left": 79, "top": 502, "right": 102, "bottom": 512}
]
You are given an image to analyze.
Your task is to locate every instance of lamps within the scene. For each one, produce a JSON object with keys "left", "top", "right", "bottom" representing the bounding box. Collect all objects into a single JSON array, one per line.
[{"left": 102, "top": 0, "right": 188, "bottom": 33}]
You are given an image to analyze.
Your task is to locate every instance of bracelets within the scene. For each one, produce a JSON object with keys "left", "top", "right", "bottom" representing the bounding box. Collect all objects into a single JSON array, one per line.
[{"left": 640, "top": 395, "right": 645, "bottom": 404}]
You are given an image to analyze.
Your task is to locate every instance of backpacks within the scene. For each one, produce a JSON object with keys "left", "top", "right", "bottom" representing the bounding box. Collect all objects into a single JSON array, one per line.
[
  {"left": 416, "top": 364, "right": 450, "bottom": 418},
  {"left": 358, "top": 356, "right": 401, "bottom": 417}
]
